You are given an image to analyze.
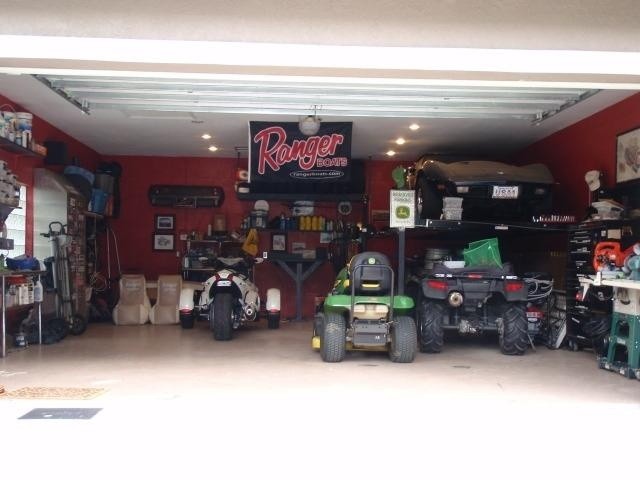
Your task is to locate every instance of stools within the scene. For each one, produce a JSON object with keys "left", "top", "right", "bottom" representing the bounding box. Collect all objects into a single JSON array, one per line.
[{"left": 603, "top": 278, "right": 640, "bottom": 374}]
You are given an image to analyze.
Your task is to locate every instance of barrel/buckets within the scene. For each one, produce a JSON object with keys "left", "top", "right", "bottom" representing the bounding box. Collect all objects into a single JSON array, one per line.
[
  {"left": 251, "top": 210, "right": 268, "bottom": 228},
  {"left": 16, "top": 112, "right": 33, "bottom": 144},
  {"left": 0, "top": 110, "right": 16, "bottom": 139}
]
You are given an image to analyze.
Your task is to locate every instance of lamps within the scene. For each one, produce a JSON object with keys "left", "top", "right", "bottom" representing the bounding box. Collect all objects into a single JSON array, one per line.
[{"left": 299, "top": 114, "right": 321, "bottom": 136}]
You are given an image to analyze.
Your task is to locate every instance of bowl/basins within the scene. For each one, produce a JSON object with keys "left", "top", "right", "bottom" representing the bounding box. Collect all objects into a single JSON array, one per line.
[{"left": 443, "top": 260, "right": 465, "bottom": 269}]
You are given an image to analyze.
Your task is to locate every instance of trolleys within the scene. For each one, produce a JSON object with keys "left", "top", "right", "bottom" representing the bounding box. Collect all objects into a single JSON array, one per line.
[{"left": 45, "top": 221, "right": 86, "bottom": 339}]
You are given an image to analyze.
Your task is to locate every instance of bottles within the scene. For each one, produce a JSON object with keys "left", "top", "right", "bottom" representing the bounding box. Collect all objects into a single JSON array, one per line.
[
  {"left": 5, "top": 274, "right": 44, "bottom": 308},
  {"left": 279, "top": 213, "right": 323, "bottom": 231}
]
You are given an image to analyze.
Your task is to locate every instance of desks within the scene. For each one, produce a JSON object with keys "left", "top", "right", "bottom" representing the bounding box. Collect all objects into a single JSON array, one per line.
[{"left": 0, "top": 265, "right": 48, "bottom": 358}]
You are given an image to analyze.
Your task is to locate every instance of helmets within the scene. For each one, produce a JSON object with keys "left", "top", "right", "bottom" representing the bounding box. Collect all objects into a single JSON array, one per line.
[
  {"left": 253, "top": 199, "right": 270, "bottom": 211},
  {"left": 6, "top": 254, "right": 40, "bottom": 271}
]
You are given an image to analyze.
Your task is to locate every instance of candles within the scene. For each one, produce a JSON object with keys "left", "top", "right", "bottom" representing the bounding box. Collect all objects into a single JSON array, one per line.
[{"left": 208, "top": 223, "right": 211, "bottom": 236}]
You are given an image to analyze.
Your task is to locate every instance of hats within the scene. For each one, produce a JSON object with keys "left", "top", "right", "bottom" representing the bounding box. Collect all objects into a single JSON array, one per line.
[{"left": 584, "top": 170, "right": 601, "bottom": 192}]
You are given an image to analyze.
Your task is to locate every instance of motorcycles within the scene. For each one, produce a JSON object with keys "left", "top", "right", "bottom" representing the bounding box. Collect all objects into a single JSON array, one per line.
[{"left": 178, "top": 248, "right": 282, "bottom": 341}]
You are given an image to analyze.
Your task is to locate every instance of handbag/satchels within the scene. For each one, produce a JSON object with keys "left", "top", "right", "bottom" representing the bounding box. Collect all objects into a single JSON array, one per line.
[{"left": 462, "top": 237, "right": 504, "bottom": 269}]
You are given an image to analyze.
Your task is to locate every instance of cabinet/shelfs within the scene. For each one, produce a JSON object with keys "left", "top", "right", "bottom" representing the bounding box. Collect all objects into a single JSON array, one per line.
[
  {"left": 34, "top": 168, "right": 104, "bottom": 323},
  {"left": 263, "top": 250, "right": 326, "bottom": 319},
  {"left": 0, "top": 134, "right": 47, "bottom": 232},
  {"left": 237, "top": 186, "right": 369, "bottom": 232},
  {"left": 373, "top": 216, "right": 570, "bottom": 315},
  {"left": 184, "top": 233, "right": 255, "bottom": 321},
  {"left": 566, "top": 215, "right": 640, "bottom": 354}
]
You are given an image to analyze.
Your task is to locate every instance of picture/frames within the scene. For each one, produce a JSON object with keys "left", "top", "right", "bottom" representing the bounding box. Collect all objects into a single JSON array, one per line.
[
  {"left": 151, "top": 233, "right": 176, "bottom": 252},
  {"left": 271, "top": 232, "right": 287, "bottom": 252},
  {"left": 615, "top": 126, "right": 640, "bottom": 185},
  {"left": 154, "top": 213, "right": 174, "bottom": 232}
]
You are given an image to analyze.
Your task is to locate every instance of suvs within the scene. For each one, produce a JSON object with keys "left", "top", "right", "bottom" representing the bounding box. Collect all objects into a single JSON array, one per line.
[
  {"left": 406, "top": 246, "right": 528, "bottom": 355},
  {"left": 312, "top": 251, "right": 417, "bottom": 363}
]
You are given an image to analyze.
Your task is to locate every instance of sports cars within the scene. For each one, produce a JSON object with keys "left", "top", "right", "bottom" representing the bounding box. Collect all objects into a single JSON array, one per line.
[{"left": 414, "top": 153, "right": 554, "bottom": 220}]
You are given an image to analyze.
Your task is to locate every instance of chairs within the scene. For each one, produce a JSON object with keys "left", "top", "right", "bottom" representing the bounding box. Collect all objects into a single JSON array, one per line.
[
  {"left": 150, "top": 273, "right": 183, "bottom": 325},
  {"left": 112, "top": 274, "right": 151, "bottom": 325},
  {"left": 344, "top": 252, "right": 390, "bottom": 296}
]
